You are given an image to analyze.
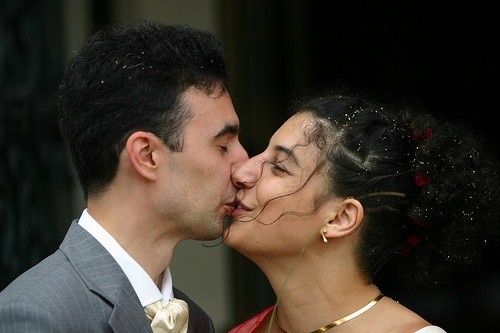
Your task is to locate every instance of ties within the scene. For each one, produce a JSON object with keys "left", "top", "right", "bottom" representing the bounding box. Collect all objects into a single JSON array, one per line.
[{"left": 142, "top": 298, "right": 190, "bottom": 333}]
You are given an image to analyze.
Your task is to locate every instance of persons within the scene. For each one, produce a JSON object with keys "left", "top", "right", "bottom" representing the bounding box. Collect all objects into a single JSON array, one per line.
[
  {"left": 1, "top": 21, "right": 249, "bottom": 332},
  {"left": 223, "top": 95, "right": 499, "bottom": 333}
]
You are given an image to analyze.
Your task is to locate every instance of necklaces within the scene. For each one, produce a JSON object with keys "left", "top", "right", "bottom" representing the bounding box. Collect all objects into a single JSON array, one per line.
[{"left": 272, "top": 292, "right": 384, "bottom": 333}]
